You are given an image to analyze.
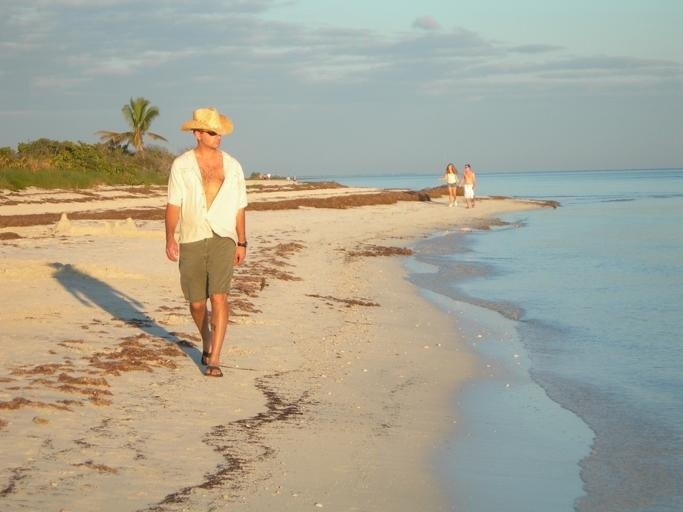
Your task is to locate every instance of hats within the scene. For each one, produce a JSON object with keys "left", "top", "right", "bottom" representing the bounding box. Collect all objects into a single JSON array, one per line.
[{"left": 181, "top": 106, "right": 233, "bottom": 134}]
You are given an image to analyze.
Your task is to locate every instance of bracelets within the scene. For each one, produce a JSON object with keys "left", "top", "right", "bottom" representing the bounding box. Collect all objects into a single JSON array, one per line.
[{"left": 236, "top": 240, "right": 247, "bottom": 247}]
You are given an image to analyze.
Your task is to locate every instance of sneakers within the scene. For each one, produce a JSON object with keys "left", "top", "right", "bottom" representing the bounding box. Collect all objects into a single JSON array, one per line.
[{"left": 449, "top": 201, "right": 457, "bottom": 208}]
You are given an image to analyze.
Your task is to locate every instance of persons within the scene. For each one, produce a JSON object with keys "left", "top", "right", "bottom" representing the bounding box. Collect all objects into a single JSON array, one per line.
[
  {"left": 458, "top": 163, "right": 477, "bottom": 208},
  {"left": 440, "top": 162, "right": 460, "bottom": 208},
  {"left": 161, "top": 105, "right": 249, "bottom": 377}
]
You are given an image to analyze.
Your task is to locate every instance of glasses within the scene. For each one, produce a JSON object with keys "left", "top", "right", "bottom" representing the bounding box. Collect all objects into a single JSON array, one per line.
[{"left": 200, "top": 130, "right": 216, "bottom": 136}]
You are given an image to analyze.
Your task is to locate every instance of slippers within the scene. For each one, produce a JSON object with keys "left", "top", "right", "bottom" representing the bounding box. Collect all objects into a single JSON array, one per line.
[{"left": 202, "top": 351, "right": 223, "bottom": 377}]
